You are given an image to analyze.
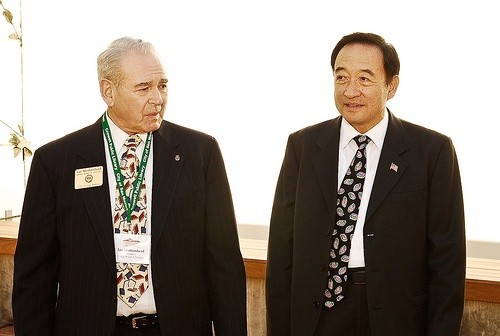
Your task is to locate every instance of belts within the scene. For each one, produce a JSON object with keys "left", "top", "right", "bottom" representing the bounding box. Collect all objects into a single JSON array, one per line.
[
  {"left": 348, "top": 269, "right": 367, "bottom": 286},
  {"left": 115, "top": 315, "right": 160, "bottom": 332}
]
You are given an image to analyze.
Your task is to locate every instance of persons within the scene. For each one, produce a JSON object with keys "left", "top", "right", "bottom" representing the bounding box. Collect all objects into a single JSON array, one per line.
[
  {"left": 12, "top": 36, "right": 249, "bottom": 336},
  {"left": 266, "top": 32, "right": 467, "bottom": 336}
]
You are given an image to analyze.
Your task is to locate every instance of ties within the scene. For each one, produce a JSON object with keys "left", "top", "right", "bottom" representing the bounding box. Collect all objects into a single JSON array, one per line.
[
  {"left": 325, "top": 134, "right": 372, "bottom": 309},
  {"left": 113, "top": 136, "right": 150, "bottom": 308}
]
